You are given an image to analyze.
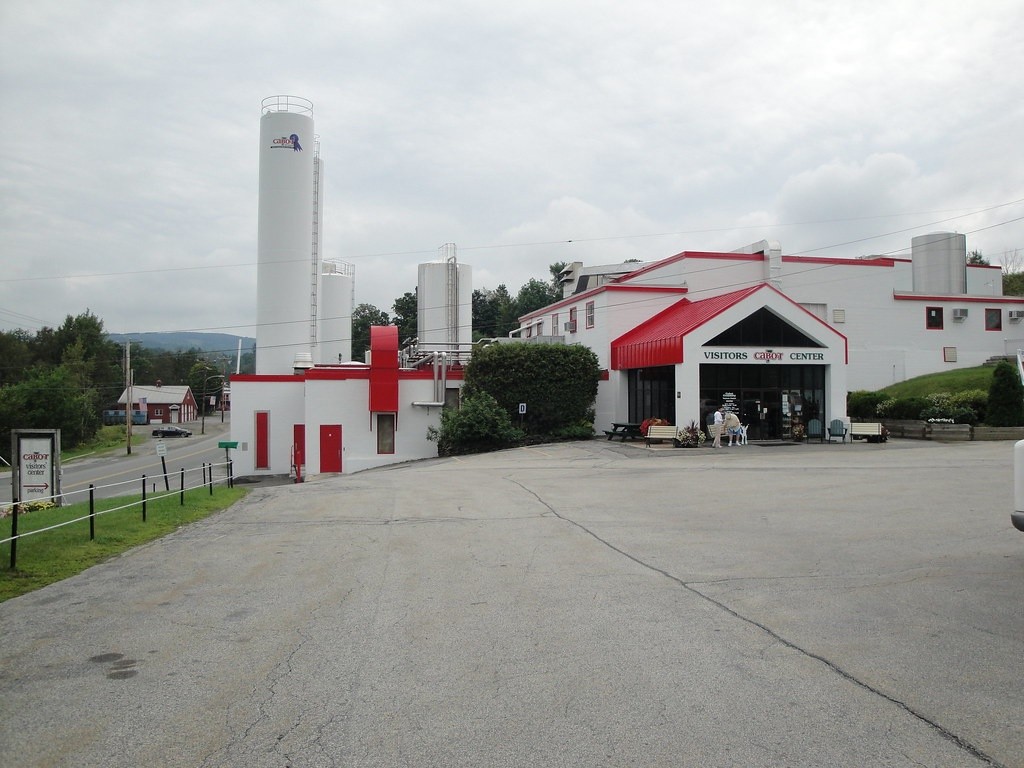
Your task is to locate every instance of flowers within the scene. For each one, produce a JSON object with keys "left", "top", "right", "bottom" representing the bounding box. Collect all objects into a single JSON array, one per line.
[
  {"left": 675, "top": 420, "right": 706, "bottom": 446},
  {"left": 639, "top": 417, "right": 670, "bottom": 436},
  {"left": 869, "top": 426, "right": 890, "bottom": 441},
  {"left": 791, "top": 424, "right": 805, "bottom": 436}
]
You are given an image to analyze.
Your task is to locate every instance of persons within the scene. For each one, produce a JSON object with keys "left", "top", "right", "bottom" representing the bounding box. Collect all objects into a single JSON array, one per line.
[
  {"left": 710, "top": 406, "right": 724, "bottom": 448},
  {"left": 724, "top": 413, "right": 741, "bottom": 446}
]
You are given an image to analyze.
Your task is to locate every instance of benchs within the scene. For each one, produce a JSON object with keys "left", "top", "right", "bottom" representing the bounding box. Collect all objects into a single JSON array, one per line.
[
  {"left": 602, "top": 430, "right": 621, "bottom": 435},
  {"left": 849, "top": 423, "right": 882, "bottom": 444},
  {"left": 644, "top": 426, "right": 678, "bottom": 448},
  {"left": 707, "top": 425, "right": 738, "bottom": 446}
]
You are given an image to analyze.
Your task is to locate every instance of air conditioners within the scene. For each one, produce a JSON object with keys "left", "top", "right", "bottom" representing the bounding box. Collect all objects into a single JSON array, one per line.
[
  {"left": 1009, "top": 311, "right": 1024, "bottom": 318},
  {"left": 953, "top": 308, "right": 969, "bottom": 318},
  {"left": 564, "top": 322, "right": 575, "bottom": 331}
]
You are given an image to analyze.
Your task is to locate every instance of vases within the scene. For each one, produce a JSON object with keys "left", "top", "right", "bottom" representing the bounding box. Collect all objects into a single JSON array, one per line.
[
  {"left": 792, "top": 434, "right": 804, "bottom": 442},
  {"left": 867, "top": 435, "right": 886, "bottom": 443},
  {"left": 681, "top": 440, "right": 689, "bottom": 448},
  {"left": 673, "top": 438, "right": 680, "bottom": 448},
  {"left": 649, "top": 438, "right": 664, "bottom": 444},
  {"left": 691, "top": 440, "right": 699, "bottom": 447}
]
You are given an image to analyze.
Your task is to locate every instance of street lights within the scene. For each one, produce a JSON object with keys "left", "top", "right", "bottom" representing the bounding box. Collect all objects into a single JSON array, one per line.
[{"left": 202, "top": 375, "right": 225, "bottom": 434}]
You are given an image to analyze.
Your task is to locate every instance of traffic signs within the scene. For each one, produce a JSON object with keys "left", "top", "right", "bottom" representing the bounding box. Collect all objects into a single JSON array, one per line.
[{"left": 11, "top": 428, "right": 61, "bottom": 504}]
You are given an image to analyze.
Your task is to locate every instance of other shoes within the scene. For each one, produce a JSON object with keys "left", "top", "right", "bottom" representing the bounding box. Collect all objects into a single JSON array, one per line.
[
  {"left": 735, "top": 442, "right": 741, "bottom": 446},
  {"left": 712, "top": 444, "right": 723, "bottom": 448},
  {"left": 728, "top": 443, "right": 733, "bottom": 446}
]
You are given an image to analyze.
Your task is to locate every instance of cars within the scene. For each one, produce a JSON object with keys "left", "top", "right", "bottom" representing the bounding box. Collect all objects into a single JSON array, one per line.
[{"left": 152, "top": 425, "right": 192, "bottom": 438}]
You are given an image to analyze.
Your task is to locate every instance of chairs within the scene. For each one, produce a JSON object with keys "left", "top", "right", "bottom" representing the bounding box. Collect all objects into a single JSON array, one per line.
[
  {"left": 805, "top": 419, "right": 825, "bottom": 444},
  {"left": 827, "top": 419, "right": 848, "bottom": 444}
]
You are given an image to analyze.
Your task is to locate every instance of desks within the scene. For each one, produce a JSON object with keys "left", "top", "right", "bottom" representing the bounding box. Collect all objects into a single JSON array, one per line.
[{"left": 607, "top": 423, "right": 644, "bottom": 442}]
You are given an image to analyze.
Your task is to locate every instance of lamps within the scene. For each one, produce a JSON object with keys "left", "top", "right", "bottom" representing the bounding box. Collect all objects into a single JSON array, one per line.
[{"left": 677, "top": 392, "right": 681, "bottom": 398}]
